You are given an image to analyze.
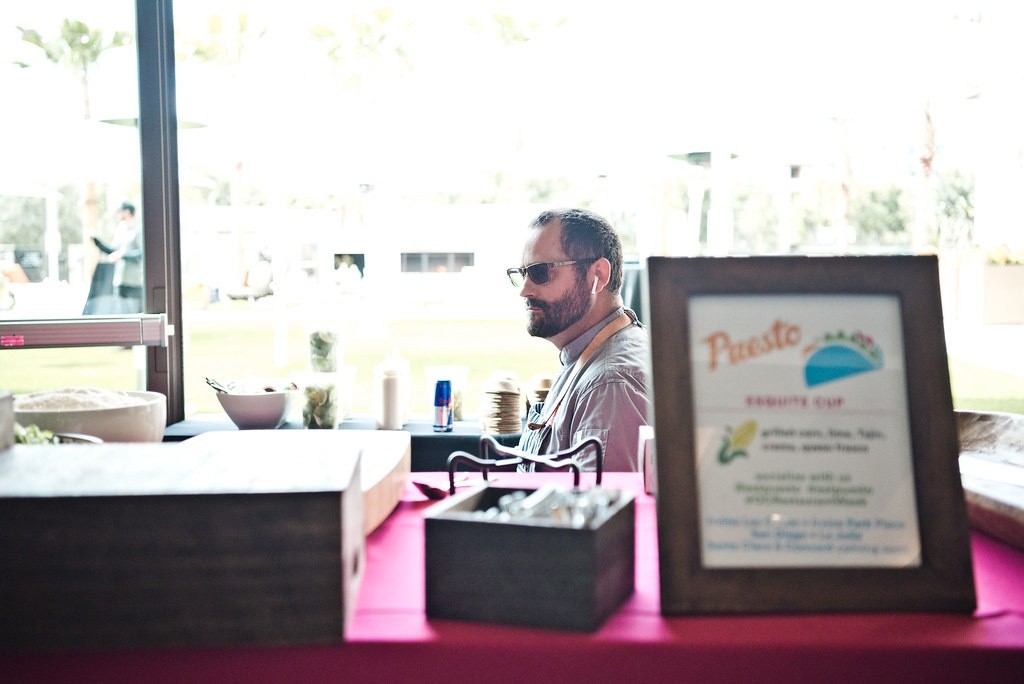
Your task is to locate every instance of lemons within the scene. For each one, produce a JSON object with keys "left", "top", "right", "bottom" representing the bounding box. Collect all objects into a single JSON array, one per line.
[{"left": 300, "top": 329, "right": 339, "bottom": 430}]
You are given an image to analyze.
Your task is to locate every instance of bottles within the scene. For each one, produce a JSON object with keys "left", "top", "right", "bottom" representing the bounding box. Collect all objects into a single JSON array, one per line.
[{"left": 382, "top": 370, "right": 402, "bottom": 430}]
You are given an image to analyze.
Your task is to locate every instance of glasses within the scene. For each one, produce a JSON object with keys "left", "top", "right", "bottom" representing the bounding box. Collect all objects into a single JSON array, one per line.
[{"left": 506, "top": 259, "right": 596, "bottom": 289}]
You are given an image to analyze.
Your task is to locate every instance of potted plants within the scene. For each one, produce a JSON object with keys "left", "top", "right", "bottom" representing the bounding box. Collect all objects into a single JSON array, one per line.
[{"left": 300, "top": 330, "right": 345, "bottom": 430}]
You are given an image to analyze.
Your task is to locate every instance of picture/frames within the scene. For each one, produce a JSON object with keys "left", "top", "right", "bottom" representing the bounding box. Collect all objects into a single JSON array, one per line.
[{"left": 644, "top": 254, "right": 978, "bottom": 616}]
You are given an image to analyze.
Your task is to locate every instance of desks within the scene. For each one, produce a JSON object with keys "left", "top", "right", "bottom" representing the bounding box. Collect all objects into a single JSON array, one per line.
[{"left": 0, "top": 468, "right": 1024, "bottom": 684}]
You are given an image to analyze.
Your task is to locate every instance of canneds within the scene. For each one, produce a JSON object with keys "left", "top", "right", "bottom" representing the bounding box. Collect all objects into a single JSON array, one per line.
[{"left": 431, "top": 380, "right": 454, "bottom": 433}]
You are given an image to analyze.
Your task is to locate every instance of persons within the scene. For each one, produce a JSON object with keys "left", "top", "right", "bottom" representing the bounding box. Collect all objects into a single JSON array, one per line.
[
  {"left": 109, "top": 203, "right": 143, "bottom": 315},
  {"left": 506, "top": 206, "right": 651, "bottom": 472}
]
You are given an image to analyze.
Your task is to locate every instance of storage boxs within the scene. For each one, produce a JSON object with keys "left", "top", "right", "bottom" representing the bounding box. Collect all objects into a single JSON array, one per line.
[{"left": 0, "top": 446, "right": 368, "bottom": 644}]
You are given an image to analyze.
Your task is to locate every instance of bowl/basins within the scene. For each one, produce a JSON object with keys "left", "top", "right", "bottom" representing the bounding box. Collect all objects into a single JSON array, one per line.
[
  {"left": 485, "top": 378, "right": 522, "bottom": 435},
  {"left": 13, "top": 391, "right": 167, "bottom": 442},
  {"left": 216, "top": 392, "right": 286, "bottom": 428}
]
